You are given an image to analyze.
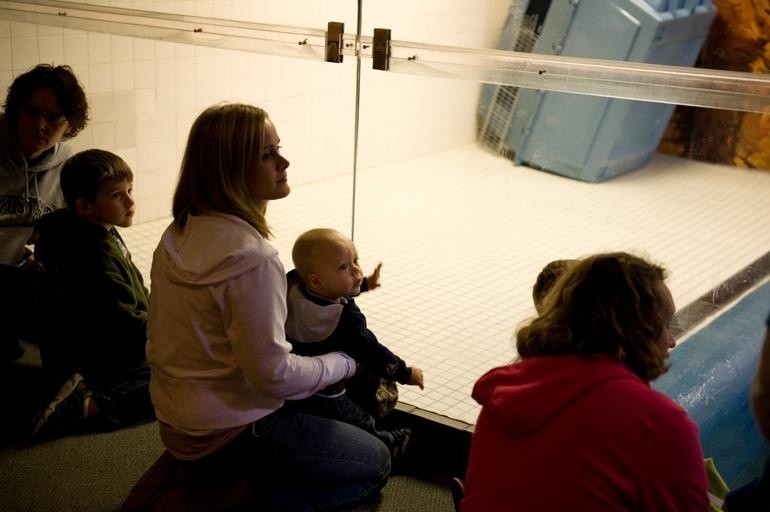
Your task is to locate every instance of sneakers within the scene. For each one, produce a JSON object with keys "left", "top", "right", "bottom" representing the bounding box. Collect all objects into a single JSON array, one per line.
[{"left": 34, "top": 372, "right": 91, "bottom": 434}]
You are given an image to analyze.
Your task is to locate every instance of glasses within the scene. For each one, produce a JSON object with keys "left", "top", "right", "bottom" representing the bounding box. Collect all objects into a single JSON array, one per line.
[{"left": 18, "top": 104, "right": 69, "bottom": 126}]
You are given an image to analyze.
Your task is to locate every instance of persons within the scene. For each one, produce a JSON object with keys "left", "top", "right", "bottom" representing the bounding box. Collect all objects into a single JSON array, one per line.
[
  {"left": 16, "top": 150, "right": 156, "bottom": 440},
  {"left": 119, "top": 101, "right": 395, "bottom": 508},
  {"left": 459, "top": 253, "right": 714, "bottom": 509},
  {"left": 1, "top": 61, "right": 94, "bottom": 275},
  {"left": 282, "top": 228, "right": 425, "bottom": 458}
]
